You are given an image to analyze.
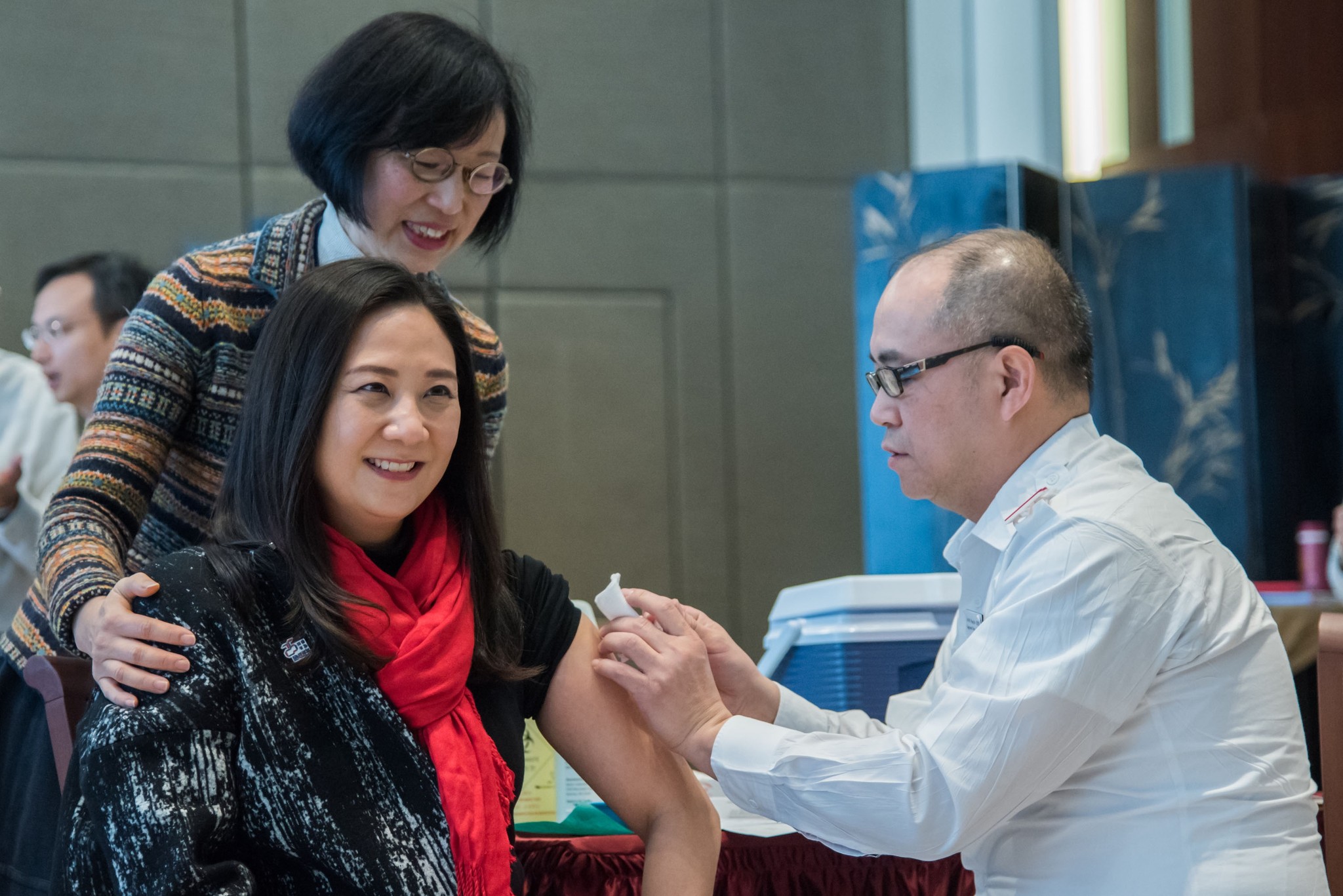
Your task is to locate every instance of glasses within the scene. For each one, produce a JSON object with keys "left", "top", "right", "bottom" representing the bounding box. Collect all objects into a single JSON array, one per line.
[
  {"left": 863, "top": 331, "right": 1046, "bottom": 399},
  {"left": 396, "top": 144, "right": 516, "bottom": 197},
  {"left": 19, "top": 314, "right": 105, "bottom": 353}
]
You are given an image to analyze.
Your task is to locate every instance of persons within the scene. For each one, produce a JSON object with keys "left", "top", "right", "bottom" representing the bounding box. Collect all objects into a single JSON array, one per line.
[
  {"left": 1, "top": 6, "right": 541, "bottom": 896},
  {"left": 47, "top": 254, "right": 723, "bottom": 895},
  {"left": 18, "top": 246, "right": 160, "bottom": 444},
  {"left": 0, "top": 346, "right": 82, "bottom": 641},
  {"left": 587, "top": 225, "right": 1335, "bottom": 896}
]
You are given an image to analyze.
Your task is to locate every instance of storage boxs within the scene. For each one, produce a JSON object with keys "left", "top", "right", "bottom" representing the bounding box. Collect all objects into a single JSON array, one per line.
[{"left": 743, "top": 569, "right": 969, "bottom": 730}]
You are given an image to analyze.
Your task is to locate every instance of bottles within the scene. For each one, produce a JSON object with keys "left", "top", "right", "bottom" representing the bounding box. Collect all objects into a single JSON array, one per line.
[{"left": 1298, "top": 519, "right": 1333, "bottom": 591}]
